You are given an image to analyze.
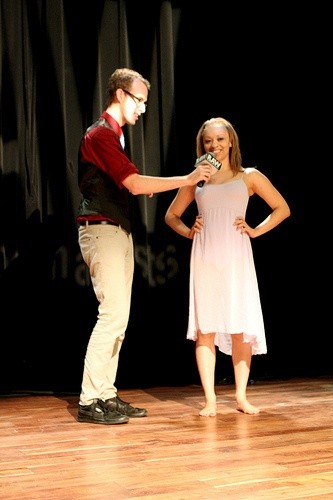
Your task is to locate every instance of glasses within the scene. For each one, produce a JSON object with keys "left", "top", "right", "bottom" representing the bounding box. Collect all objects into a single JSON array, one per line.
[{"left": 123, "top": 90, "right": 148, "bottom": 107}]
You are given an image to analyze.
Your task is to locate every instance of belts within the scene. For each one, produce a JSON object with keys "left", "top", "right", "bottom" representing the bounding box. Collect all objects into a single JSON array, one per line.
[{"left": 80, "top": 220, "right": 119, "bottom": 226}]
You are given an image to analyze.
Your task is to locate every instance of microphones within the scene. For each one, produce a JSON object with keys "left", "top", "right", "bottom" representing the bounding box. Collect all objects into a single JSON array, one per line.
[{"left": 194, "top": 152, "right": 222, "bottom": 188}]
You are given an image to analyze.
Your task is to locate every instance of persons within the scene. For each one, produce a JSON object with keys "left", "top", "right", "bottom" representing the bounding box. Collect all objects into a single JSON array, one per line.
[
  {"left": 165, "top": 118, "right": 290, "bottom": 416},
  {"left": 76, "top": 69, "right": 212, "bottom": 424}
]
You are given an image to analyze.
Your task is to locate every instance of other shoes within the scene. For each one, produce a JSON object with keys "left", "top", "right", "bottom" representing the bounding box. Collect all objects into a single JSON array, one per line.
[{"left": 78, "top": 395, "right": 147, "bottom": 424}]
